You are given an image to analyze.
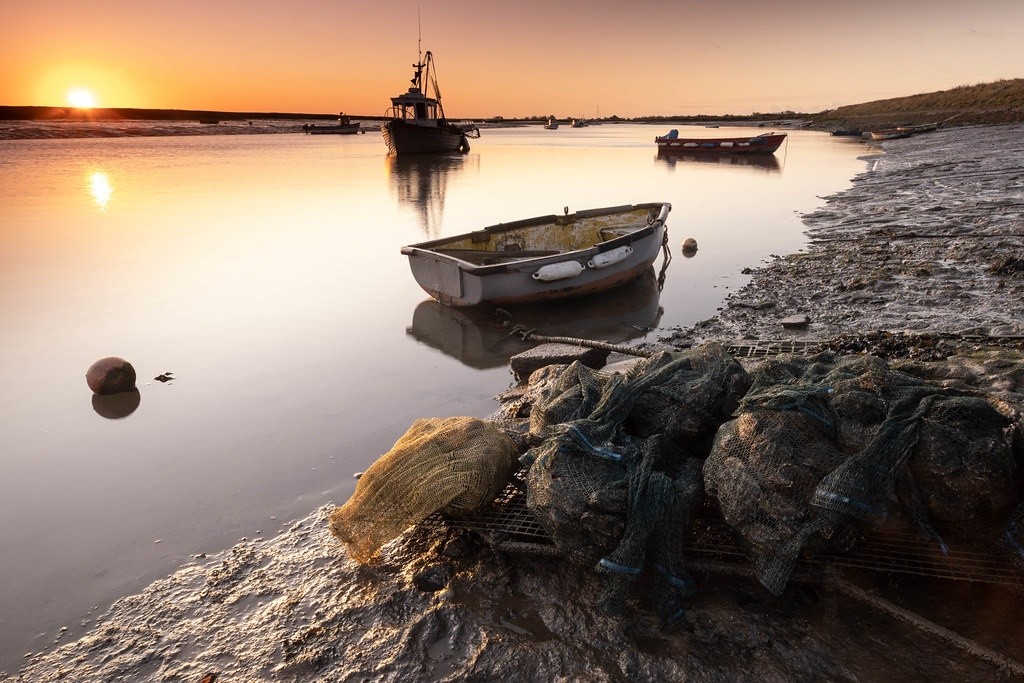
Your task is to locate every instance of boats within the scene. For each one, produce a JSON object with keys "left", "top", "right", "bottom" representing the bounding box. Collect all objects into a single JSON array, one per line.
[
  {"left": 570, "top": 104, "right": 602, "bottom": 127},
  {"left": 302, "top": 112, "right": 361, "bottom": 134},
  {"left": 870, "top": 127, "right": 916, "bottom": 141},
  {"left": 378, "top": 6, "right": 475, "bottom": 155},
  {"left": 400, "top": 201, "right": 673, "bottom": 309},
  {"left": 655, "top": 128, "right": 788, "bottom": 155},
  {"left": 543, "top": 119, "right": 558, "bottom": 129},
  {"left": 897, "top": 121, "right": 938, "bottom": 134}
]
